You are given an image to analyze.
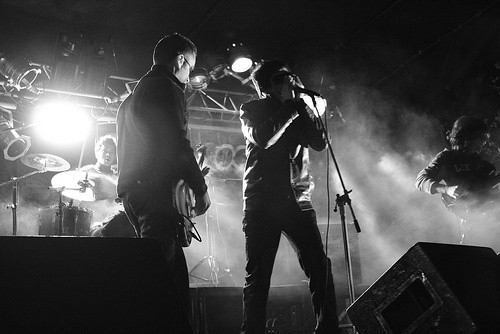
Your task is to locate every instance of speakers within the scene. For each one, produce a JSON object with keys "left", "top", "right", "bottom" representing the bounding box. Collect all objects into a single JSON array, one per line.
[
  {"left": 0, "top": 237, "right": 195, "bottom": 334},
  {"left": 347, "top": 242, "right": 500, "bottom": 334}
]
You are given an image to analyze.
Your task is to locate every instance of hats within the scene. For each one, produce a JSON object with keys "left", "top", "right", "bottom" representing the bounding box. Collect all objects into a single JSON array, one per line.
[{"left": 253, "top": 60, "right": 288, "bottom": 93}]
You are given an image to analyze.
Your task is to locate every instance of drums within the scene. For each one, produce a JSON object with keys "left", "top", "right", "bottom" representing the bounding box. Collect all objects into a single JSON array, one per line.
[{"left": 38, "top": 205, "right": 93, "bottom": 236}]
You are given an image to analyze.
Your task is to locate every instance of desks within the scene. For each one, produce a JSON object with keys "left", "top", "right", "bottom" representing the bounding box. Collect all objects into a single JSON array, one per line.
[{"left": 0, "top": 232, "right": 183, "bottom": 334}]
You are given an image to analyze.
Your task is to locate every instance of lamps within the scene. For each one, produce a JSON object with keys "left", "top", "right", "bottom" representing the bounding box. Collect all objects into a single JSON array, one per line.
[
  {"left": 230, "top": 44, "right": 265, "bottom": 86},
  {"left": 187, "top": 56, "right": 211, "bottom": 91}
]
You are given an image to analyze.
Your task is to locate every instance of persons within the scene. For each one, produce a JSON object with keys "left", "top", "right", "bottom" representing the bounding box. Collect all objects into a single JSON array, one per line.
[
  {"left": 415, "top": 115, "right": 500, "bottom": 254},
  {"left": 79, "top": 135, "right": 125, "bottom": 229},
  {"left": 239, "top": 59, "right": 349, "bottom": 334},
  {"left": 117, "top": 32, "right": 210, "bottom": 326}
]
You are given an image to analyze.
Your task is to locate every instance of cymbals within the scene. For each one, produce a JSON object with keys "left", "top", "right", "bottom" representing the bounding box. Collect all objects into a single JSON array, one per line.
[
  {"left": 21, "top": 153, "right": 70, "bottom": 172},
  {"left": 50, "top": 170, "right": 117, "bottom": 202}
]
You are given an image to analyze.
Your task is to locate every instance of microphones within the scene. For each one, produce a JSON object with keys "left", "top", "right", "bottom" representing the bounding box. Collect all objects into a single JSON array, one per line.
[{"left": 294, "top": 86, "right": 322, "bottom": 96}]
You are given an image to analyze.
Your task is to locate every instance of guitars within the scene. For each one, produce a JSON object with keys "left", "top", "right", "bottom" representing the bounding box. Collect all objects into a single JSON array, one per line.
[
  {"left": 441, "top": 171, "right": 500, "bottom": 222},
  {"left": 171, "top": 144, "right": 207, "bottom": 248}
]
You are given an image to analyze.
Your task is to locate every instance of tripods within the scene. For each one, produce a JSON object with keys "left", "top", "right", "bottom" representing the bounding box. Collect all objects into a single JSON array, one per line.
[{"left": 187, "top": 210, "right": 237, "bottom": 288}]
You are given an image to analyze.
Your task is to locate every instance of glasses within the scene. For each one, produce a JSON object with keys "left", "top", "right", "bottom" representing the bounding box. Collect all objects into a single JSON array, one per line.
[{"left": 177, "top": 53, "right": 195, "bottom": 74}]
